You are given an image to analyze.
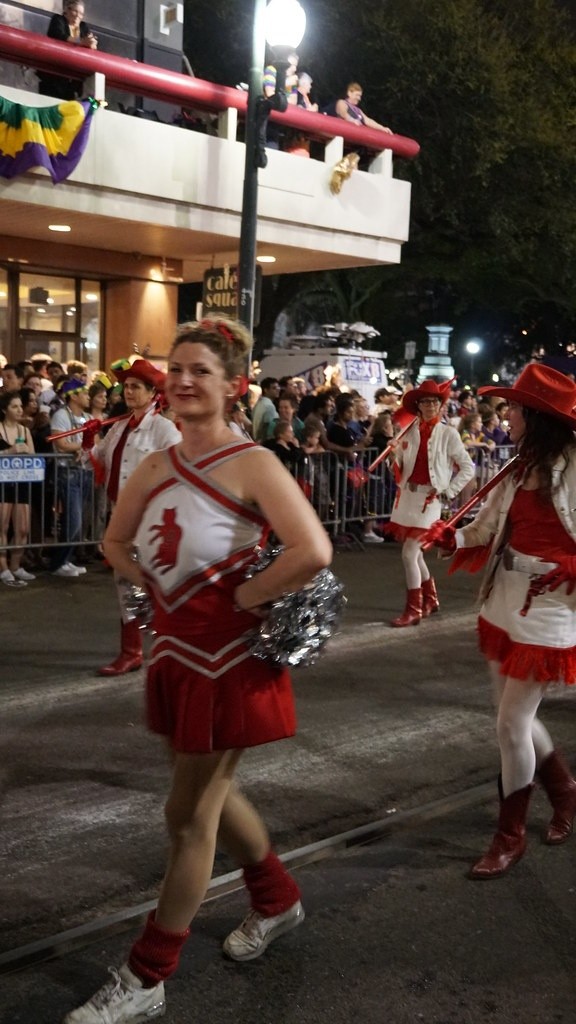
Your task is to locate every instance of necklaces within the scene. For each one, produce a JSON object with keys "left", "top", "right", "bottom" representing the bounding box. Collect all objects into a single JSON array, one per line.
[{"left": 3, "top": 422, "right": 19, "bottom": 445}]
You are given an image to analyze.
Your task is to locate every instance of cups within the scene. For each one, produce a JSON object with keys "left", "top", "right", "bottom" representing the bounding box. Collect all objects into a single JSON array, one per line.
[{"left": 15, "top": 438, "right": 25, "bottom": 443}]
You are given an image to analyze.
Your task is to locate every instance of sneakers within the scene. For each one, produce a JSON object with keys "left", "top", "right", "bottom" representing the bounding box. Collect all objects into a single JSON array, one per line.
[
  {"left": 223, "top": 896, "right": 305, "bottom": 962},
  {"left": 65, "top": 964, "right": 165, "bottom": 1024},
  {"left": 51, "top": 562, "right": 87, "bottom": 577},
  {"left": 13, "top": 568, "right": 36, "bottom": 579},
  {"left": 363, "top": 530, "right": 384, "bottom": 542},
  {"left": 3, "top": 579, "right": 28, "bottom": 587}
]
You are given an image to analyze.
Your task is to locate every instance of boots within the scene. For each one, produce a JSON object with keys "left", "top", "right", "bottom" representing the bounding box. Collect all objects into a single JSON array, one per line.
[
  {"left": 534, "top": 750, "right": 576, "bottom": 846},
  {"left": 98, "top": 616, "right": 144, "bottom": 675},
  {"left": 469, "top": 773, "right": 535, "bottom": 879},
  {"left": 422, "top": 576, "right": 440, "bottom": 617},
  {"left": 390, "top": 588, "right": 423, "bottom": 626}
]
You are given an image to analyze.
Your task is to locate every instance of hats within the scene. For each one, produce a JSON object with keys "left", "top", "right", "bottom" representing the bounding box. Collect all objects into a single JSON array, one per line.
[
  {"left": 110, "top": 360, "right": 166, "bottom": 394},
  {"left": 477, "top": 364, "right": 576, "bottom": 421},
  {"left": 61, "top": 378, "right": 86, "bottom": 398},
  {"left": 375, "top": 388, "right": 393, "bottom": 397},
  {"left": 403, "top": 376, "right": 456, "bottom": 416}
]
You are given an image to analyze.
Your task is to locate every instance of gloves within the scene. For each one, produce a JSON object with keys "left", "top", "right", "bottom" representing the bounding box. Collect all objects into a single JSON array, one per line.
[
  {"left": 541, "top": 555, "right": 576, "bottom": 595},
  {"left": 424, "top": 521, "right": 456, "bottom": 552},
  {"left": 81, "top": 419, "right": 102, "bottom": 450}
]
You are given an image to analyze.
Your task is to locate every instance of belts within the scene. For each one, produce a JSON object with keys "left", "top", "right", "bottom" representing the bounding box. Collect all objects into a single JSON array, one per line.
[
  {"left": 503, "top": 544, "right": 559, "bottom": 577},
  {"left": 405, "top": 481, "right": 432, "bottom": 492}
]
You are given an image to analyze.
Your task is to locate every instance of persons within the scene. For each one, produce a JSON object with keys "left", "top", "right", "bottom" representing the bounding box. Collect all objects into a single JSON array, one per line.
[
  {"left": 443, "top": 382, "right": 523, "bottom": 528},
  {"left": 252, "top": 363, "right": 414, "bottom": 543},
  {"left": 386, "top": 375, "right": 476, "bottom": 628},
  {"left": 424, "top": 363, "right": 576, "bottom": 880},
  {"left": 259, "top": 51, "right": 299, "bottom": 150},
  {"left": 335, "top": 81, "right": 394, "bottom": 157},
  {"left": 227, "top": 360, "right": 262, "bottom": 443},
  {"left": 65, "top": 310, "right": 333, "bottom": 1024},
  {"left": 559, "top": 365, "right": 574, "bottom": 381},
  {"left": 0, "top": 352, "right": 130, "bottom": 586},
  {"left": 280, "top": 71, "right": 319, "bottom": 159},
  {"left": 81, "top": 360, "right": 184, "bottom": 677},
  {"left": 32, "top": 0, "right": 98, "bottom": 100}
]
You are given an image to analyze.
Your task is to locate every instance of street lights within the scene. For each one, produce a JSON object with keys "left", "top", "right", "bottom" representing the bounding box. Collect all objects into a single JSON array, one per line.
[
  {"left": 230, "top": 1, "right": 309, "bottom": 368},
  {"left": 465, "top": 341, "right": 481, "bottom": 378}
]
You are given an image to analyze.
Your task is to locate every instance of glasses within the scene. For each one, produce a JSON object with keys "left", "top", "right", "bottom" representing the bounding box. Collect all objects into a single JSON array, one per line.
[{"left": 418, "top": 397, "right": 441, "bottom": 407}]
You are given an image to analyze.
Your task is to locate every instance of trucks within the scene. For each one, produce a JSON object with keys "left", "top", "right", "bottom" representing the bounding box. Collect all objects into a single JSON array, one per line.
[{"left": 258, "top": 346, "right": 389, "bottom": 407}]
